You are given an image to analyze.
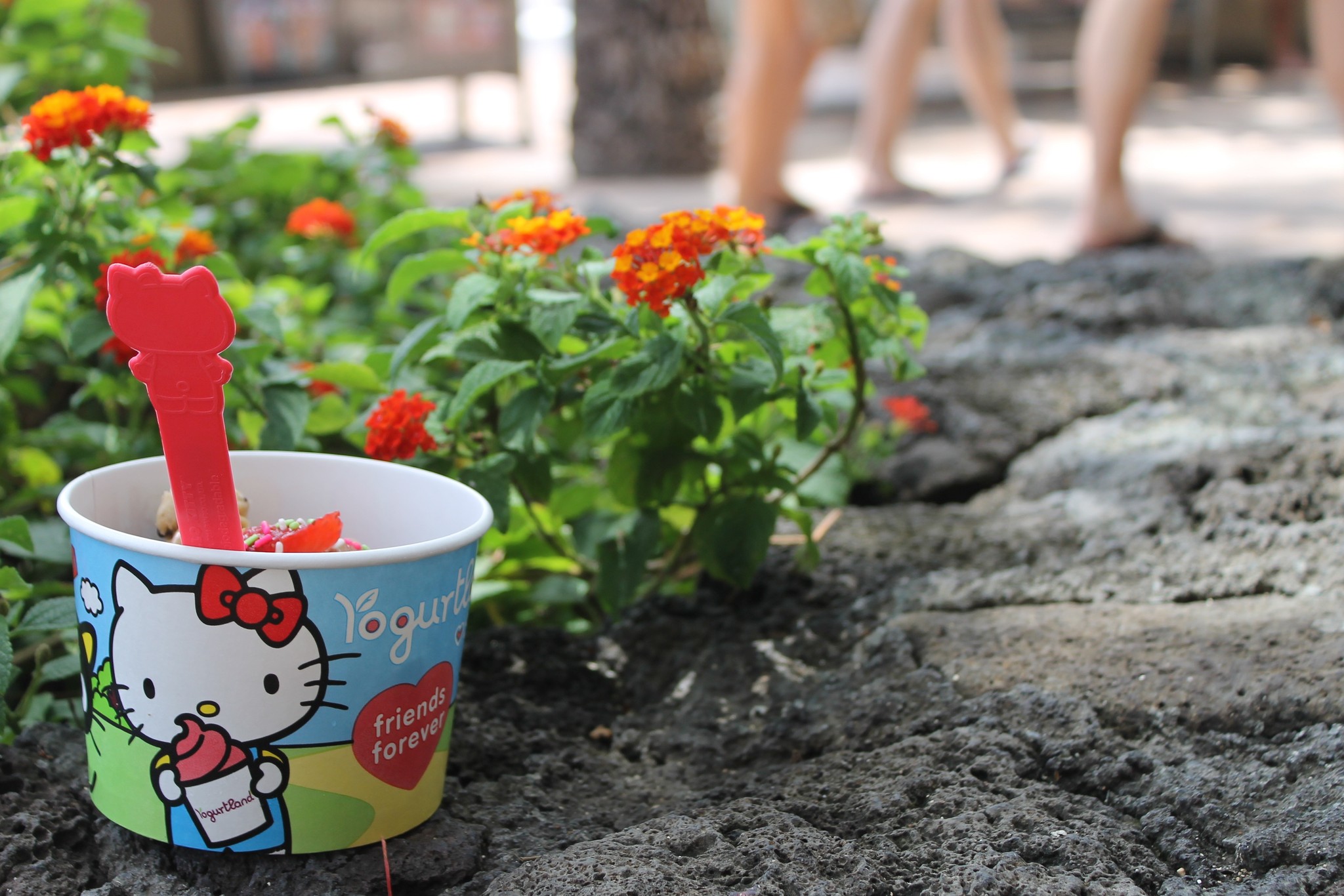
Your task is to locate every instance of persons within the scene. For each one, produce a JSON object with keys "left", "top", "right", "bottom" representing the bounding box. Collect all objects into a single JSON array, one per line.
[
  {"left": 1070, "top": 1, "right": 1344, "bottom": 251},
  {"left": 725, "top": 0, "right": 839, "bottom": 250},
  {"left": 858, "top": 3, "right": 1045, "bottom": 209}
]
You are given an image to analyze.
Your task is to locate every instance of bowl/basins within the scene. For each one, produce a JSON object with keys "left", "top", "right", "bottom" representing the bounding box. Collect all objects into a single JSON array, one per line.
[{"left": 57, "top": 450, "right": 494, "bottom": 855}]
